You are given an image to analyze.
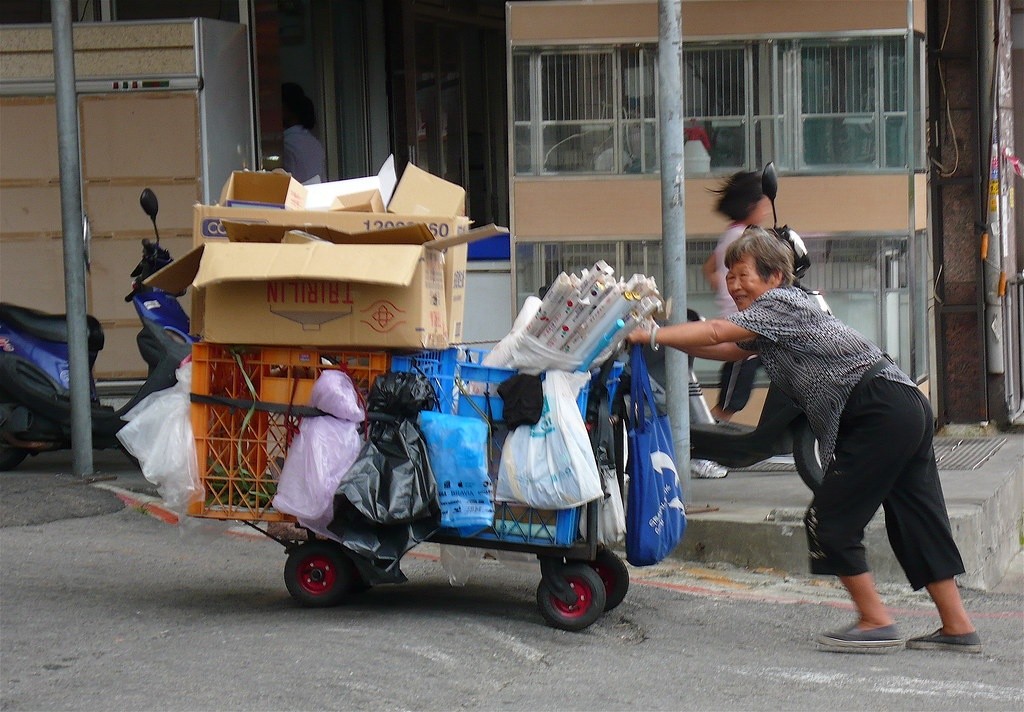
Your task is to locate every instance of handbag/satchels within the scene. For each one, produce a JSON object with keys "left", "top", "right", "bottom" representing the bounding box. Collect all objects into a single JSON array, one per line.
[
  {"left": 625, "top": 344, "right": 687, "bottom": 566},
  {"left": 495, "top": 369, "right": 605, "bottom": 510},
  {"left": 579, "top": 399, "right": 627, "bottom": 548}
]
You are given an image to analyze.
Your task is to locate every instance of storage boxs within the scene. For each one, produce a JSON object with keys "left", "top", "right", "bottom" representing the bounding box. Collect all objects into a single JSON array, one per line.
[
  {"left": 187, "top": 342, "right": 391, "bottom": 522},
  {"left": 143, "top": 154, "right": 510, "bottom": 349},
  {"left": 390, "top": 348, "right": 590, "bottom": 549}
]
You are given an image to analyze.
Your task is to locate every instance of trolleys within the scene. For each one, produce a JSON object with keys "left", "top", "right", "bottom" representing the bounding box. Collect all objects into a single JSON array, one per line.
[{"left": 186, "top": 320, "right": 630, "bottom": 632}]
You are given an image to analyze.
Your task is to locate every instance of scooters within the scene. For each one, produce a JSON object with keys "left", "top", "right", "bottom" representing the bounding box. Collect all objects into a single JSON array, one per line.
[
  {"left": 538, "top": 161, "right": 833, "bottom": 491},
  {"left": 1, "top": 188, "right": 200, "bottom": 471}
]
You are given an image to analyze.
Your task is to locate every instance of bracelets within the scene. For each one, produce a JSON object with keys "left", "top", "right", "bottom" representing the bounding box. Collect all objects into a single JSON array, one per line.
[{"left": 651, "top": 325, "right": 660, "bottom": 351}]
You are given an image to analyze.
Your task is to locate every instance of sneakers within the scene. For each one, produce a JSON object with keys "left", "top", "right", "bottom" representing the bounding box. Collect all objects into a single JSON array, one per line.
[
  {"left": 817, "top": 623, "right": 905, "bottom": 654},
  {"left": 906, "top": 627, "right": 985, "bottom": 653},
  {"left": 690, "top": 458, "right": 730, "bottom": 478}
]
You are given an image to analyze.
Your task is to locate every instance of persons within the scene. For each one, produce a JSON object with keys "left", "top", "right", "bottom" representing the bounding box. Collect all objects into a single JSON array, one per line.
[
  {"left": 624, "top": 228, "right": 982, "bottom": 654},
  {"left": 690, "top": 170, "right": 769, "bottom": 478},
  {"left": 279, "top": 81, "right": 327, "bottom": 185}
]
